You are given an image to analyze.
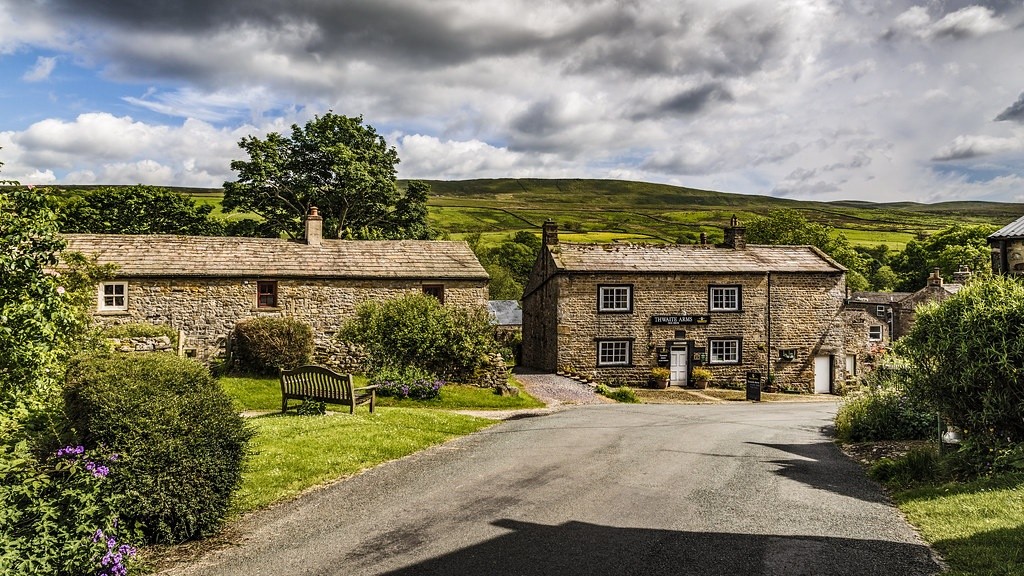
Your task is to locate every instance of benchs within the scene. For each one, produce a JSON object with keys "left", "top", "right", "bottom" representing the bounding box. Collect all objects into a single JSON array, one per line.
[{"left": 278, "top": 365, "right": 379, "bottom": 414}]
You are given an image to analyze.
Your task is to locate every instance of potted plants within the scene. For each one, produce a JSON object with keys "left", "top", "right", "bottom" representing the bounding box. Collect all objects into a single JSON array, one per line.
[
  {"left": 652, "top": 367, "right": 671, "bottom": 389},
  {"left": 765, "top": 375, "right": 778, "bottom": 393},
  {"left": 692, "top": 367, "right": 713, "bottom": 389}
]
